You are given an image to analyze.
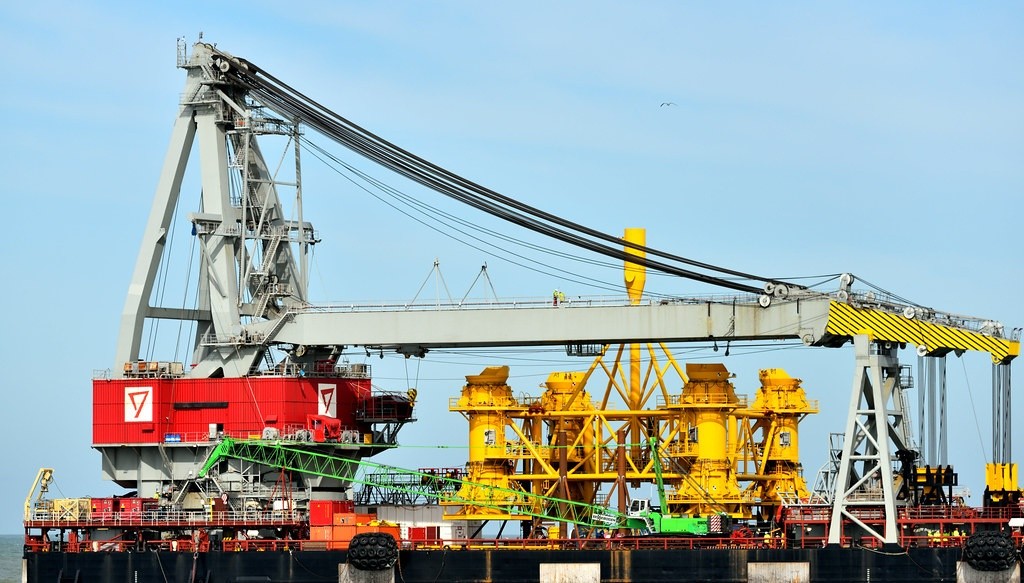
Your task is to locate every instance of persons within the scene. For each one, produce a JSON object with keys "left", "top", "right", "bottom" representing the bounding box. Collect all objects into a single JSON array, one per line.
[
  {"left": 754, "top": 527, "right": 786, "bottom": 549},
  {"left": 41, "top": 545, "right": 48, "bottom": 552},
  {"left": 154, "top": 491, "right": 161, "bottom": 500},
  {"left": 552, "top": 289, "right": 559, "bottom": 306},
  {"left": 928, "top": 527, "right": 966, "bottom": 548},
  {"left": 557, "top": 292, "right": 565, "bottom": 304}
]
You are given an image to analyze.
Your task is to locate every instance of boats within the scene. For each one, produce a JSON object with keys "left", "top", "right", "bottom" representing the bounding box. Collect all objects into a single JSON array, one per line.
[{"left": 21, "top": 31, "right": 1024, "bottom": 582}]
